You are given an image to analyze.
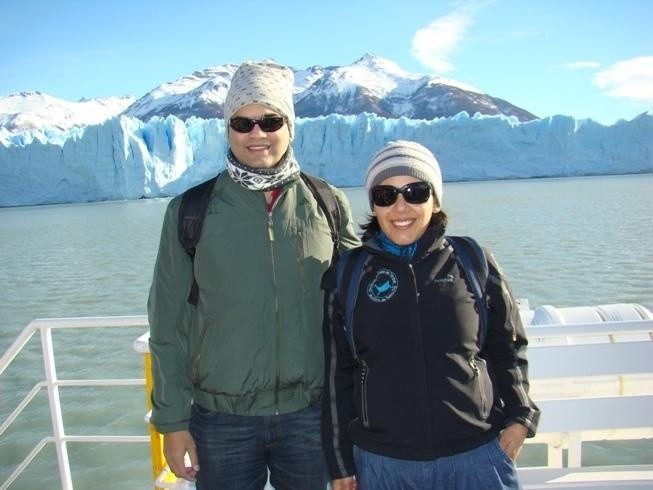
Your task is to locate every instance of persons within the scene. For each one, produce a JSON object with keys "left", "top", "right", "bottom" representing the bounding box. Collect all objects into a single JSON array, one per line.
[
  {"left": 147, "top": 61, "right": 362, "bottom": 490},
  {"left": 319, "top": 139, "right": 541, "bottom": 489}
]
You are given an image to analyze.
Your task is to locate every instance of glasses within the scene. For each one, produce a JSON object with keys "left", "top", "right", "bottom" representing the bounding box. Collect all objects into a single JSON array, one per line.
[
  {"left": 371, "top": 182, "right": 432, "bottom": 206},
  {"left": 230, "top": 116, "right": 284, "bottom": 133}
]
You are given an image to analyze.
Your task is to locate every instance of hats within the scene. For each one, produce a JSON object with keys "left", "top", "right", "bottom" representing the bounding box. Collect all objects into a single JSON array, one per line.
[
  {"left": 364, "top": 140, "right": 443, "bottom": 206},
  {"left": 225, "top": 62, "right": 295, "bottom": 140}
]
